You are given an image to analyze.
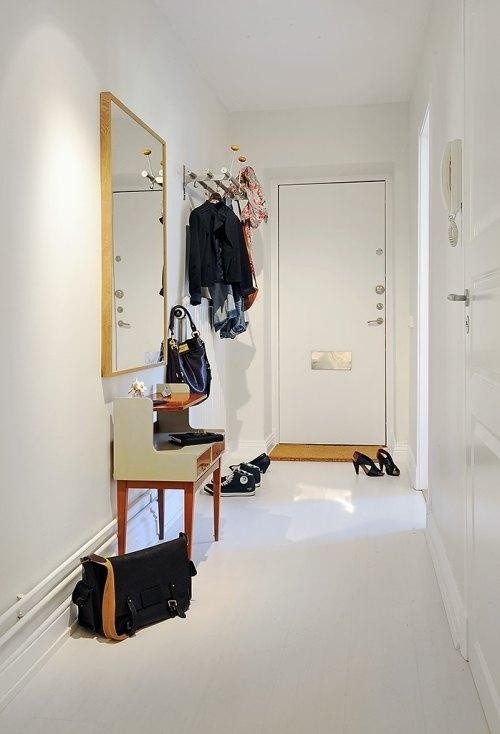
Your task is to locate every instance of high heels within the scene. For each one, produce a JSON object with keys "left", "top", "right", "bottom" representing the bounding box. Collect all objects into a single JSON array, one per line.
[
  {"left": 229, "top": 453, "right": 270, "bottom": 473},
  {"left": 351, "top": 451, "right": 385, "bottom": 476},
  {"left": 376, "top": 448, "right": 400, "bottom": 476}
]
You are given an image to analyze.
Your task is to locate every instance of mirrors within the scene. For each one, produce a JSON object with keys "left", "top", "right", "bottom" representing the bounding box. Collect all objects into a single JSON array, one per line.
[{"left": 99, "top": 92, "right": 168, "bottom": 374}]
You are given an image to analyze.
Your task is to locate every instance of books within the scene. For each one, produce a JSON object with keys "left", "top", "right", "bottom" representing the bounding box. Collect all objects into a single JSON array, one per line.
[{"left": 167, "top": 432, "right": 223, "bottom": 447}]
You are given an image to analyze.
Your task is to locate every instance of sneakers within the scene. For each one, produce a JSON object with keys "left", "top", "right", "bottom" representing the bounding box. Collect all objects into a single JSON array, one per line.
[{"left": 204, "top": 461, "right": 260, "bottom": 497}]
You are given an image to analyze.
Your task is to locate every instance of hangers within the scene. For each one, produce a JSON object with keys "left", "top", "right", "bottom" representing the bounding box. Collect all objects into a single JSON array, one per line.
[{"left": 189, "top": 181, "right": 240, "bottom": 222}]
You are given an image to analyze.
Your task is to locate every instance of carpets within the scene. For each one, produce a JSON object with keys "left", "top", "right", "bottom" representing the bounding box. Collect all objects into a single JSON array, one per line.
[{"left": 269, "top": 444, "right": 384, "bottom": 463}]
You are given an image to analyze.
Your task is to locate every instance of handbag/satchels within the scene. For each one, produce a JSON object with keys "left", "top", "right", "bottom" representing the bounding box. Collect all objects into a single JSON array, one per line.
[
  {"left": 73, "top": 531, "right": 197, "bottom": 641},
  {"left": 168, "top": 334, "right": 211, "bottom": 406},
  {"left": 244, "top": 288, "right": 258, "bottom": 311}
]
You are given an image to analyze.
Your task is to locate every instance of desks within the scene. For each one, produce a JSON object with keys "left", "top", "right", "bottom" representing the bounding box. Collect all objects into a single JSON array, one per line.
[{"left": 113, "top": 393, "right": 225, "bottom": 560}]
